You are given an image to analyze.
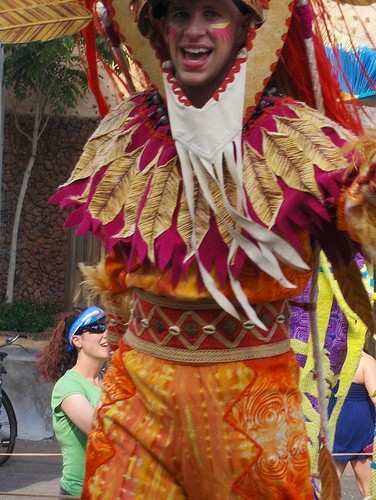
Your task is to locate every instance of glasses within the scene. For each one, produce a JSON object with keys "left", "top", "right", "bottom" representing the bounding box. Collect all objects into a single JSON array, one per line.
[{"left": 75, "top": 322, "right": 106, "bottom": 335}]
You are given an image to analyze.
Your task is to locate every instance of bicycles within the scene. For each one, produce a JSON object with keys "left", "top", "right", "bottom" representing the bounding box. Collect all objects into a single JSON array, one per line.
[{"left": 0, "top": 334, "right": 28, "bottom": 466}]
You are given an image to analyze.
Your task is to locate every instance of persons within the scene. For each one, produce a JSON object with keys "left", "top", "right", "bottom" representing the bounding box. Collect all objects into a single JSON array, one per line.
[
  {"left": 46, "top": 1, "right": 376, "bottom": 500},
  {"left": 36, "top": 305, "right": 110, "bottom": 497},
  {"left": 317, "top": 348, "right": 375, "bottom": 500}
]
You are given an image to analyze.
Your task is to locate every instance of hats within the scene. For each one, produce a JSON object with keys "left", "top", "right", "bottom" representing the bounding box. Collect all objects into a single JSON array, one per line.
[{"left": 133, "top": 0, "right": 266, "bottom": 34}]
